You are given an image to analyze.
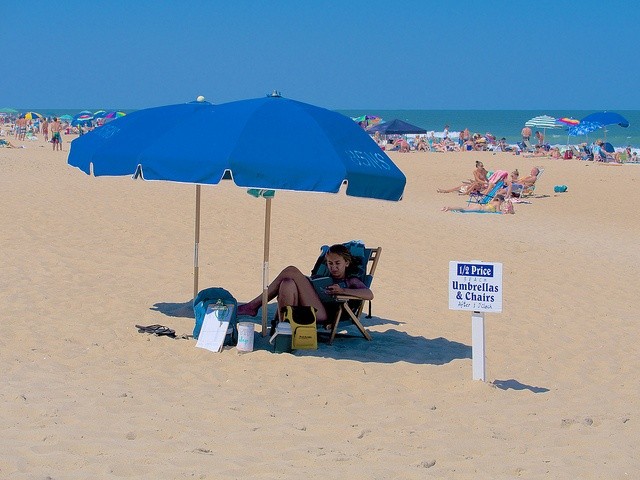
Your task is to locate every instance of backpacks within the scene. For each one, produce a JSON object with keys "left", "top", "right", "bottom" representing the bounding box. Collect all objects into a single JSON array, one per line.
[{"left": 193, "top": 287, "right": 239, "bottom": 345}]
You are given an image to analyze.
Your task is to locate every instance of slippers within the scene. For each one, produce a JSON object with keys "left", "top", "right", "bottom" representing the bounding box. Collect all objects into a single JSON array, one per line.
[
  {"left": 136, "top": 324, "right": 166, "bottom": 332},
  {"left": 146, "top": 325, "right": 175, "bottom": 338}
]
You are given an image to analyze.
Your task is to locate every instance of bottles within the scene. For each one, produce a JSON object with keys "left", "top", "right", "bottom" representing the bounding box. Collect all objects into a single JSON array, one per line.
[{"left": 237, "top": 322, "right": 254, "bottom": 352}]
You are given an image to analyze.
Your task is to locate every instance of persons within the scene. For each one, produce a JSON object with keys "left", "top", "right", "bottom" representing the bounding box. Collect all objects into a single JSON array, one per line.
[
  {"left": 373, "top": 130, "right": 382, "bottom": 146},
  {"left": 437, "top": 168, "right": 488, "bottom": 193},
  {"left": 412, "top": 126, "right": 519, "bottom": 151},
  {"left": 495, "top": 167, "right": 540, "bottom": 199},
  {"left": 522, "top": 126, "right": 533, "bottom": 149},
  {"left": 537, "top": 140, "right": 639, "bottom": 163},
  {"left": 479, "top": 169, "right": 521, "bottom": 196},
  {"left": 1, "top": 115, "right": 63, "bottom": 151},
  {"left": 463, "top": 161, "right": 489, "bottom": 194},
  {"left": 441, "top": 194, "right": 504, "bottom": 213}
]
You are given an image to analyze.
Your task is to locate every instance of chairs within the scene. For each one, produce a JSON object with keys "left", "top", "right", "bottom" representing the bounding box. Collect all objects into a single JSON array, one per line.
[
  {"left": 508, "top": 166, "right": 544, "bottom": 199},
  {"left": 460, "top": 170, "right": 508, "bottom": 194},
  {"left": 269, "top": 247, "right": 382, "bottom": 345},
  {"left": 466, "top": 181, "right": 504, "bottom": 208}
]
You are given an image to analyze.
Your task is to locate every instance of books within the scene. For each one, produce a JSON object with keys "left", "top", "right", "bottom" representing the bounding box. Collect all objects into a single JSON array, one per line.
[
  {"left": 305, "top": 275, "right": 332, "bottom": 302},
  {"left": 194, "top": 304, "right": 233, "bottom": 352}
]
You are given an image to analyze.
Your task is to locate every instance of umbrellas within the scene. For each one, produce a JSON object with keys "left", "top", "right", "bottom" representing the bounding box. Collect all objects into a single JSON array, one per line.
[
  {"left": 67, "top": 96, "right": 214, "bottom": 301},
  {"left": 557, "top": 116, "right": 579, "bottom": 146},
  {"left": 525, "top": 114, "right": 558, "bottom": 146},
  {"left": 580, "top": 110, "right": 630, "bottom": 144},
  {"left": 19, "top": 108, "right": 125, "bottom": 121},
  {"left": 356, "top": 114, "right": 382, "bottom": 129},
  {"left": 569, "top": 120, "right": 606, "bottom": 145},
  {"left": 132, "top": 90, "right": 407, "bottom": 337}
]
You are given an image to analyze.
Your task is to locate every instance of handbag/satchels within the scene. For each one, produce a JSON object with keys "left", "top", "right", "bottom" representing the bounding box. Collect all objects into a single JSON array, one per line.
[{"left": 501, "top": 200, "right": 515, "bottom": 214}]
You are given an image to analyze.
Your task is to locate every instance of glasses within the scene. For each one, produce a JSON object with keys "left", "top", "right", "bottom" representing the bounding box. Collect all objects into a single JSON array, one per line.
[{"left": 478, "top": 164, "right": 483, "bottom": 167}]
